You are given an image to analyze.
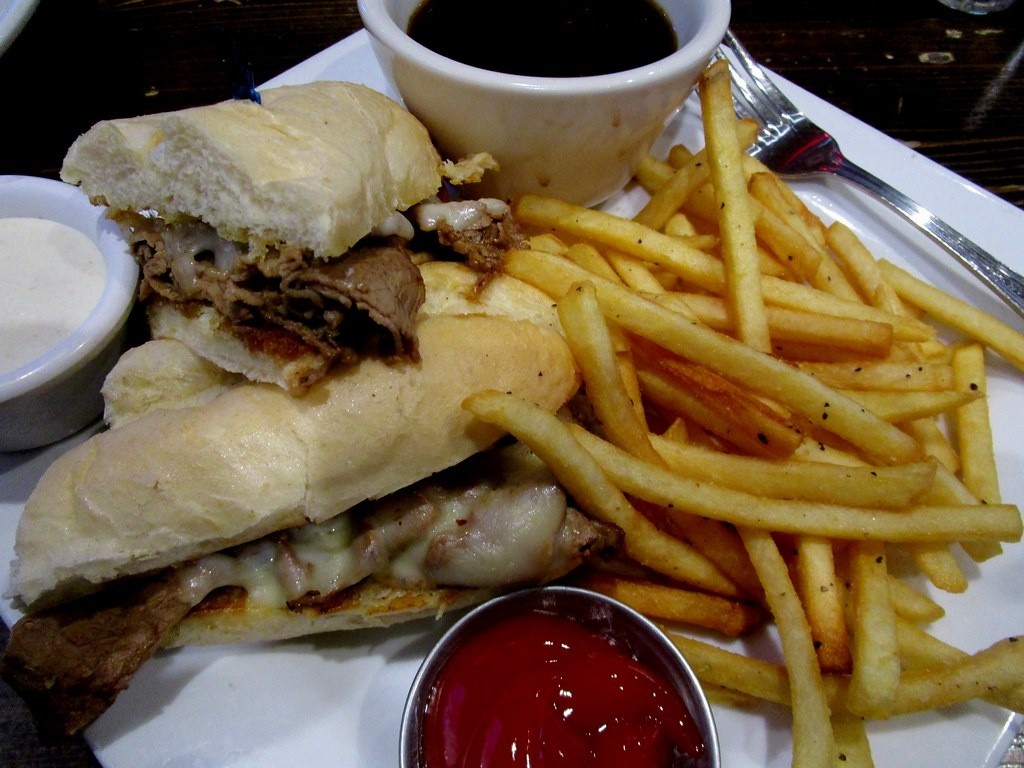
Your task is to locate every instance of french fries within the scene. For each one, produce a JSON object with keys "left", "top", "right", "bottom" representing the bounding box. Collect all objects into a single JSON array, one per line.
[{"left": 467, "top": 59, "right": 1024, "bottom": 768}]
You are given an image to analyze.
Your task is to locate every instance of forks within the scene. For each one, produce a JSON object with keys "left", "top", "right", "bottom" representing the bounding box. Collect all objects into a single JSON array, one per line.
[{"left": 693, "top": 25, "right": 1024, "bottom": 320}]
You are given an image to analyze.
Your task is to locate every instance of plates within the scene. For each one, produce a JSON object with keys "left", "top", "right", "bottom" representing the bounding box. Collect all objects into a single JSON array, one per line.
[{"left": 0, "top": 21, "right": 1024, "bottom": 768}]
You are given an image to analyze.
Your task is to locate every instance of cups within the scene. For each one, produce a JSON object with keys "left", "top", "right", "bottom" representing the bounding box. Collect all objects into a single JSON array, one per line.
[
  {"left": 357, "top": 0, "right": 733, "bottom": 210},
  {"left": 0, "top": 168, "right": 142, "bottom": 458}
]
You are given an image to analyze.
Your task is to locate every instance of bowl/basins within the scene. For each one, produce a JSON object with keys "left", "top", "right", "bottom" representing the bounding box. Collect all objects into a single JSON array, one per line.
[{"left": 398, "top": 586, "right": 720, "bottom": 768}]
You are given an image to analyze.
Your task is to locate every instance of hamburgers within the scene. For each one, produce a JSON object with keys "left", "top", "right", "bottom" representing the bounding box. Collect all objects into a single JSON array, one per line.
[{"left": 2, "top": 80, "right": 625, "bottom": 768}]
